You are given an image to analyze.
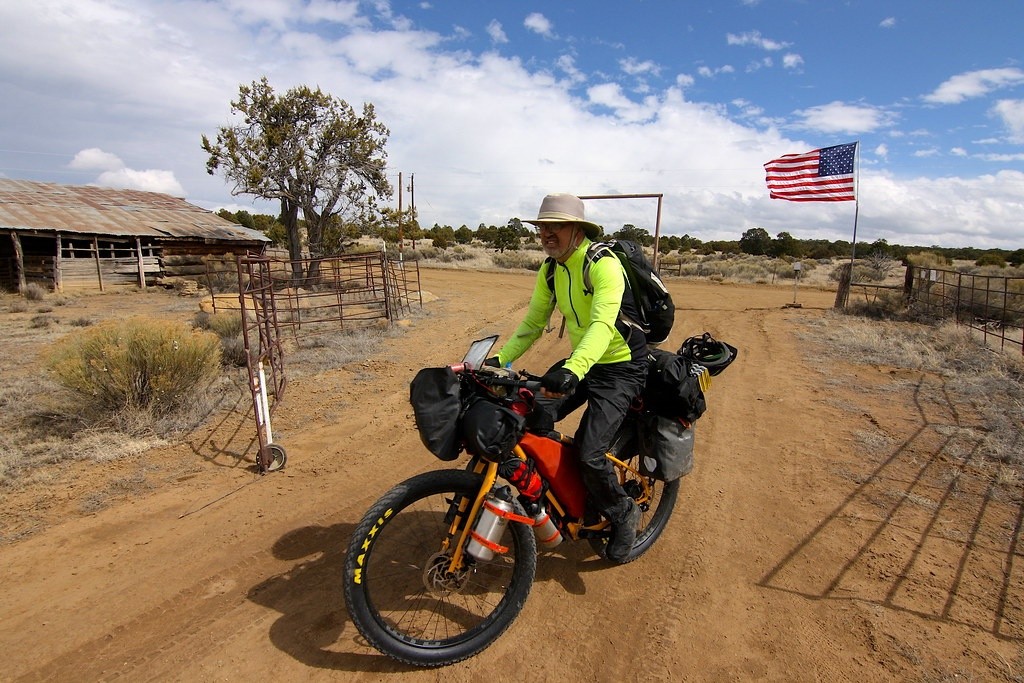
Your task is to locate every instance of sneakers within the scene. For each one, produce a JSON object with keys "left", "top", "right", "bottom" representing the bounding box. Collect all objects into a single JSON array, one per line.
[{"left": 605, "top": 497, "right": 642, "bottom": 563}]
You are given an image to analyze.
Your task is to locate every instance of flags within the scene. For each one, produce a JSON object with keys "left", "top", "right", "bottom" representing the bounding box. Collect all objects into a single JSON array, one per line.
[{"left": 764, "top": 141, "right": 856, "bottom": 202}]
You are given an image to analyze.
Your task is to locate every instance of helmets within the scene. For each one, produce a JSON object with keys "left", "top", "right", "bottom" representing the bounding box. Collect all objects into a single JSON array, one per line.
[{"left": 677, "top": 336, "right": 739, "bottom": 376}]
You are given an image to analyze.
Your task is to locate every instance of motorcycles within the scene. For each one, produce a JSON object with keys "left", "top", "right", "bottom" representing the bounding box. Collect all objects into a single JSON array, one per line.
[{"left": 340, "top": 333, "right": 739, "bottom": 670}]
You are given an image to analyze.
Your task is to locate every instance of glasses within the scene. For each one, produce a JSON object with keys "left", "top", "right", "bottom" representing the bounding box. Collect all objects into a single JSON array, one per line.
[{"left": 534, "top": 223, "right": 576, "bottom": 234}]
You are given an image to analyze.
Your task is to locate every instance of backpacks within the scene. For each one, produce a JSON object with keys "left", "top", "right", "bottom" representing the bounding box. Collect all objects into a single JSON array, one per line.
[{"left": 544, "top": 239, "right": 675, "bottom": 350}]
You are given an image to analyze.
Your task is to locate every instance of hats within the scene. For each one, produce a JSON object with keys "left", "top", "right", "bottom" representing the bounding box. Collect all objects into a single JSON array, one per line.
[{"left": 521, "top": 193, "right": 602, "bottom": 242}]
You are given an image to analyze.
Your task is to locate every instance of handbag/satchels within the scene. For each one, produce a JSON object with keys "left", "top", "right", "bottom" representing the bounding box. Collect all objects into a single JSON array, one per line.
[
  {"left": 411, "top": 367, "right": 464, "bottom": 462},
  {"left": 642, "top": 349, "right": 706, "bottom": 426},
  {"left": 463, "top": 399, "right": 524, "bottom": 463}
]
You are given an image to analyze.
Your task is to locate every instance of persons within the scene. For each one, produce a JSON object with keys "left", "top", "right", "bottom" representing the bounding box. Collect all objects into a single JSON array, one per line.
[{"left": 481, "top": 193, "right": 657, "bottom": 563}]
